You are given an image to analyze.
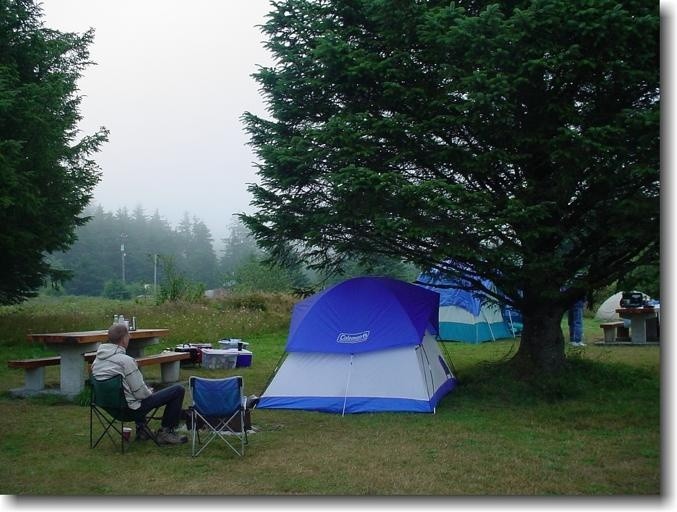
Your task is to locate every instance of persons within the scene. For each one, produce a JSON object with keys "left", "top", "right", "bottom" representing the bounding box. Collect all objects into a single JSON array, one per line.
[
  {"left": 559, "top": 268, "right": 589, "bottom": 347},
  {"left": 90, "top": 322, "right": 188, "bottom": 445}
]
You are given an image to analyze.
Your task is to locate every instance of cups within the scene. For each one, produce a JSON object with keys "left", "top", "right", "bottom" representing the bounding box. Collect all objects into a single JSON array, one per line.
[
  {"left": 122, "top": 427, "right": 131, "bottom": 443},
  {"left": 237, "top": 343, "right": 242, "bottom": 351}
]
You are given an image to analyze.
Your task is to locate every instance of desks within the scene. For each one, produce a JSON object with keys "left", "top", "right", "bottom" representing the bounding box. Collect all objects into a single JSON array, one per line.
[
  {"left": 614, "top": 306, "right": 659, "bottom": 345},
  {"left": 26, "top": 328, "right": 169, "bottom": 400}
]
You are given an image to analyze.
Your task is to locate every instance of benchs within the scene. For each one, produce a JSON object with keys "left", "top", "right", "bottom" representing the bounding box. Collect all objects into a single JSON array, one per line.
[
  {"left": 598, "top": 319, "right": 629, "bottom": 343},
  {"left": 134, "top": 349, "right": 192, "bottom": 383},
  {"left": 6, "top": 351, "right": 95, "bottom": 392}
]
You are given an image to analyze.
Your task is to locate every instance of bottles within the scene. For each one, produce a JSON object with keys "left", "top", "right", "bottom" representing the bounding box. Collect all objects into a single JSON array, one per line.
[{"left": 111, "top": 314, "right": 135, "bottom": 331}]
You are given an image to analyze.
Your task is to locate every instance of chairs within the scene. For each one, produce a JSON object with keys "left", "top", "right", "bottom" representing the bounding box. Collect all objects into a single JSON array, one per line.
[{"left": 89, "top": 372, "right": 258, "bottom": 457}]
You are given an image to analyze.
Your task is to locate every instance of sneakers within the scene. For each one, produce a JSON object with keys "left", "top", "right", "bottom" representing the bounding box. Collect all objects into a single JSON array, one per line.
[
  {"left": 152, "top": 426, "right": 188, "bottom": 445},
  {"left": 136, "top": 424, "right": 154, "bottom": 442}
]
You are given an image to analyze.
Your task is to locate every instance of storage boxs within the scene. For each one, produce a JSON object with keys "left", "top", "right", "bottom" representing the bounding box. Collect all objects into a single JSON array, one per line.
[{"left": 175, "top": 337, "right": 254, "bottom": 370}]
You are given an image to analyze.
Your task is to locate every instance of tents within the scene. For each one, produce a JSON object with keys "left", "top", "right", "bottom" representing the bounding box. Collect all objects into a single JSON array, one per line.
[
  {"left": 251, "top": 275, "right": 459, "bottom": 415},
  {"left": 592, "top": 289, "right": 651, "bottom": 321},
  {"left": 414, "top": 252, "right": 525, "bottom": 345}
]
